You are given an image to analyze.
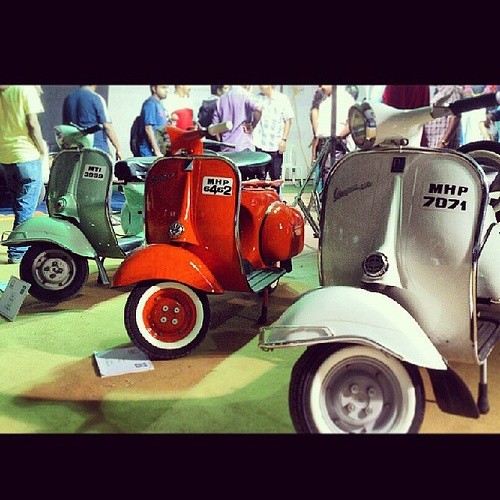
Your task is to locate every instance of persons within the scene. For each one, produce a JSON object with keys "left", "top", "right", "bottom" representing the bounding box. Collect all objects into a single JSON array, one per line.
[
  {"left": 130, "top": 84, "right": 179, "bottom": 168},
  {"left": 1, "top": 86, "right": 53, "bottom": 265},
  {"left": 193, "top": 85, "right": 294, "bottom": 207},
  {"left": 56, "top": 86, "right": 126, "bottom": 229},
  {"left": 372, "top": 85, "right": 499, "bottom": 156},
  {"left": 307, "top": 86, "right": 365, "bottom": 213}
]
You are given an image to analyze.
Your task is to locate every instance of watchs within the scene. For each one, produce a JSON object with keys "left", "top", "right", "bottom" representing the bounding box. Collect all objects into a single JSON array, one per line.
[
  {"left": 282, "top": 138, "right": 288, "bottom": 143},
  {"left": 441, "top": 142, "right": 449, "bottom": 146}
]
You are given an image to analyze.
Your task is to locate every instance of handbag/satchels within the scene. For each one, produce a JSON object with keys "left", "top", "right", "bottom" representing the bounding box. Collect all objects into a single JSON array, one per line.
[{"left": 130, "top": 116, "right": 141, "bottom": 156}]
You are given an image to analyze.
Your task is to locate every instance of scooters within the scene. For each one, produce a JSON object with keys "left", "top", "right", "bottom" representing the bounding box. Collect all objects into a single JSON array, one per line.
[
  {"left": 0, "top": 121, "right": 273, "bottom": 303},
  {"left": 255, "top": 88, "right": 500, "bottom": 435},
  {"left": 109, "top": 118, "right": 305, "bottom": 360}
]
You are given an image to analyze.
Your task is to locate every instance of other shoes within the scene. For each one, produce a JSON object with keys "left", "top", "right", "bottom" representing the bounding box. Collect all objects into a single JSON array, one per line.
[
  {"left": 111, "top": 215, "right": 121, "bottom": 225},
  {"left": 8, "top": 256, "right": 22, "bottom": 263}
]
